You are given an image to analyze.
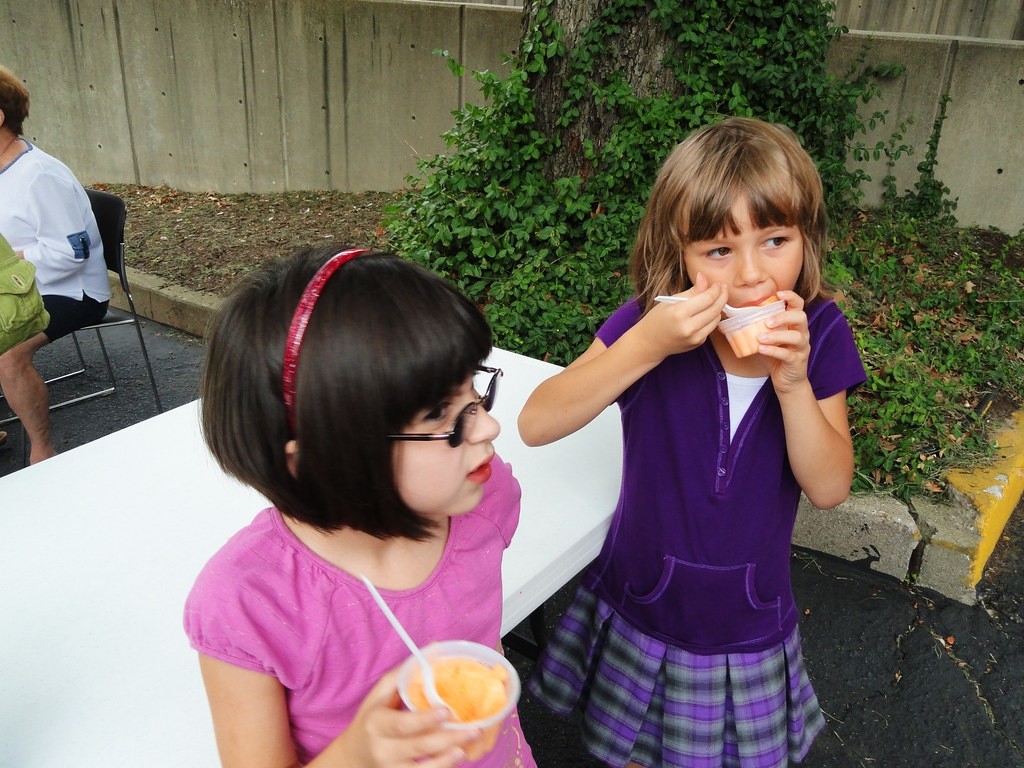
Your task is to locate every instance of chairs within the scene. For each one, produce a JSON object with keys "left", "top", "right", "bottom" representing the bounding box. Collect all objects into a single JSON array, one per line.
[{"left": 0, "top": 190, "right": 164, "bottom": 465}]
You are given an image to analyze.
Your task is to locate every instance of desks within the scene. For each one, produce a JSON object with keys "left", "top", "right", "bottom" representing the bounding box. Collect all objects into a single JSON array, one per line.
[{"left": 1, "top": 341, "right": 624, "bottom": 768}]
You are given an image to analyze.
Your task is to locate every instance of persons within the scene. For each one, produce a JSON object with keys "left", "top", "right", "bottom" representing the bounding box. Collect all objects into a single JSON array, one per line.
[
  {"left": 519, "top": 114, "right": 867, "bottom": 768},
  {"left": 184, "top": 240, "right": 538, "bottom": 768},
  {"left": 0, "top": 63, "right": 110, "bottom": 465}
]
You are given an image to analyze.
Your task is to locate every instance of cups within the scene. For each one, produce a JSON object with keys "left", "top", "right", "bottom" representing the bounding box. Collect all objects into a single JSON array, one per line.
[{"left": 398, "top": 640, "right": 520, "bottom": 762}]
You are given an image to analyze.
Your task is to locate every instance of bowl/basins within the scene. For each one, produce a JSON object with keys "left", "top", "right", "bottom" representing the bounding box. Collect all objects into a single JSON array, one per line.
[{"left": 718, "top": 300, "right": 787, "bottom": 358}]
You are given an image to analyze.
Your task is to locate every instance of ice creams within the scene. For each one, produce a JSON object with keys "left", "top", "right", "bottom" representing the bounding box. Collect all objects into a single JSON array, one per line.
[
  {"left": 397, "top": 638, "right": 520, "bottom": 760},
  {"left": 718, "top": 296, "right": 786, "bottom": 358}
]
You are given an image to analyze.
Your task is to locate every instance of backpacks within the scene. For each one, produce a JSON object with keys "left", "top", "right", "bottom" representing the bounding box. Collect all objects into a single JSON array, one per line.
[{"left": 0, "top": 235, "right": 50, "bottom": 355}]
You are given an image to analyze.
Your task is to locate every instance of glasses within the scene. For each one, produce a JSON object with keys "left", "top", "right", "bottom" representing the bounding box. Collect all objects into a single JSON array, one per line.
[{"left": 386, "top": 364, "right": 504, "bottom": 446}]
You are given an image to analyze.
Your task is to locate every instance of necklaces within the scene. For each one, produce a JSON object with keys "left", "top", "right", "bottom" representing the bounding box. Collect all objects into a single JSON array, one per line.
[{"left": 0, "top": 135, "right": 18, "bottom": 159}]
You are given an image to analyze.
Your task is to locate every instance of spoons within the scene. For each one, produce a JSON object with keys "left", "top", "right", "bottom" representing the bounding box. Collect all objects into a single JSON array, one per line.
[
  {"left": 654, "top": 296, "right": 760, "bottom": 318},
  {"left": 360, "top": 575, "right": 457, "bottom": 721}
]
